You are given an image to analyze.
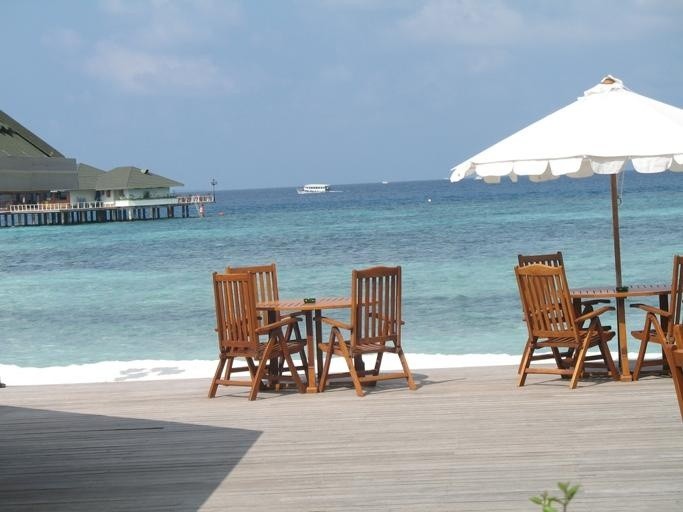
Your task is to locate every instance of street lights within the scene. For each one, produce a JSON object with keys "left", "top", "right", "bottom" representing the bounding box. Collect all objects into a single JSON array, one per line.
[{"left": 210, "top": 178, "right": 218, "bottom": 204}]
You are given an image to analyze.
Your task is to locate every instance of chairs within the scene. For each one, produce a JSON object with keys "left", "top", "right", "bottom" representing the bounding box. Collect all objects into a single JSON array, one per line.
[
  {"left": 206, "top": 266, "right": 309, "bottom": 402},
  {"left": 662, "top": 342, "right": 682, "bottom": 422},
  {"left": 626, "top": 252, "right": 681, "bottom": 385},
  {"left": 313, "top": 265, "right": 417, "bottom": 398},
  {"left": 515, "top": 250, "right": 615, "bottom": 379},
  {"left": 225, "top": 261, "right": 315, "bottom": 390},
  {"left": 511, "top": 262, "right": 620, "bottom": 390}
]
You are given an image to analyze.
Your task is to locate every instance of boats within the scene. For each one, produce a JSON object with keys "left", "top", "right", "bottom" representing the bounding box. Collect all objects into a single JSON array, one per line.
[
  {"left": 473, "top": 177, "right": 483, "bottom": 183},
  {"left": 382, "top": 181, "right": 388, "bottom": 185},
  {"left": 297, "top": 184, "right": 330, "bottom": 193}
]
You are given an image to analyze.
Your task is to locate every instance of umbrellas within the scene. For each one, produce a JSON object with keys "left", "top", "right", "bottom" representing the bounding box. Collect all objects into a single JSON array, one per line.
[{"left": 450, "top": 74, "right": 683, "bottom": 287}]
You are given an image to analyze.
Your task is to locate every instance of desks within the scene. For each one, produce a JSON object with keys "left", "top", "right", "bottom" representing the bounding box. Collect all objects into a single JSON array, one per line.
[
  {"left": 252, "top": 295, "right": 379, "bottom": 392},
  {"left": 559, "top": 282, "right": 681, "bottom": 384}
]
